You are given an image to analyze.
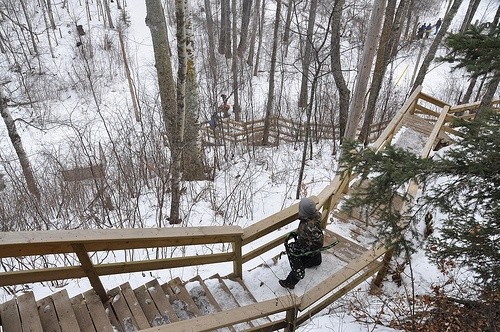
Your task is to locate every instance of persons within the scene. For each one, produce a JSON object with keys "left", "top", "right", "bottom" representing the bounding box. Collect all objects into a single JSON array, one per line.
[
  {"left": 209, "top": 112, "right": 217, "bottom": 130},
  {"left": 434, "top": 18, "right": 441, "bottom": 35},
  {"left": 278, "top": 198, "right": 324, "bottom": 289},
  {"left": 221, "top": 94, "right": 228, "bottom": 109},
  {"left": 425, "top": 23, "right": 432, "bottom": 38},
  {"left": 417, "top": 23, "right": 426, "bottom": 40}
]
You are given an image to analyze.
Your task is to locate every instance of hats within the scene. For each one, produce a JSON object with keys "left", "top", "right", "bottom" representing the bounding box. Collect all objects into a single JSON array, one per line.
[{"left": 299, "top": 197, "right": 317, "bottom": 217}]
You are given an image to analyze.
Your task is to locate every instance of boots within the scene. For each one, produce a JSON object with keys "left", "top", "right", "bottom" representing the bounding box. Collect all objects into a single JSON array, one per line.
[{"left": 278, "top": 271, "right": 300, "bottom": 290}]
